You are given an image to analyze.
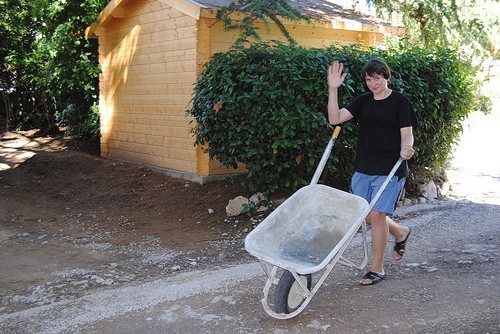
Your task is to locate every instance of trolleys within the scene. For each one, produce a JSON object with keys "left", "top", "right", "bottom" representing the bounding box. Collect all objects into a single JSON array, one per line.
[{"left": 244, "top": 126, "right": 411, "bottom": 319}]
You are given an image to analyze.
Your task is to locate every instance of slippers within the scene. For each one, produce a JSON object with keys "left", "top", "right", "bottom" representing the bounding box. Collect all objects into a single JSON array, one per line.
[
  {"left": 359, "top": 271, "right": 386, "bottom": 285},
  {"left": 392, "top": 225, "right": 411, "bottom": 261}
]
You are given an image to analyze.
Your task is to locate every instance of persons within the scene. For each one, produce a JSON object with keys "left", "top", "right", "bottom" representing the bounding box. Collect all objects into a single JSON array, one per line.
[{"left": 327, "top": 59, "right": 415, "bottom": 286}]
